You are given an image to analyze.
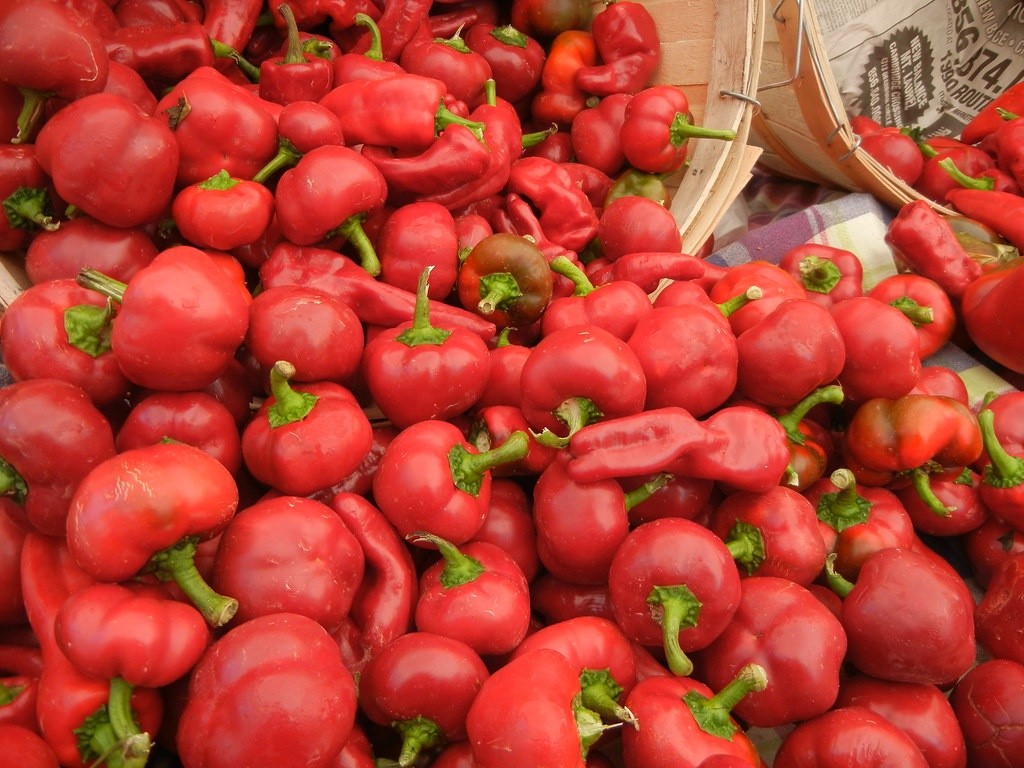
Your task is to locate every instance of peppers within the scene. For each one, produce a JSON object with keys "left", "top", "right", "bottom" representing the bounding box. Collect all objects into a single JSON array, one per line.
[{"left": 0, "top": 0, "right": 1024, "bottom": 768}]
